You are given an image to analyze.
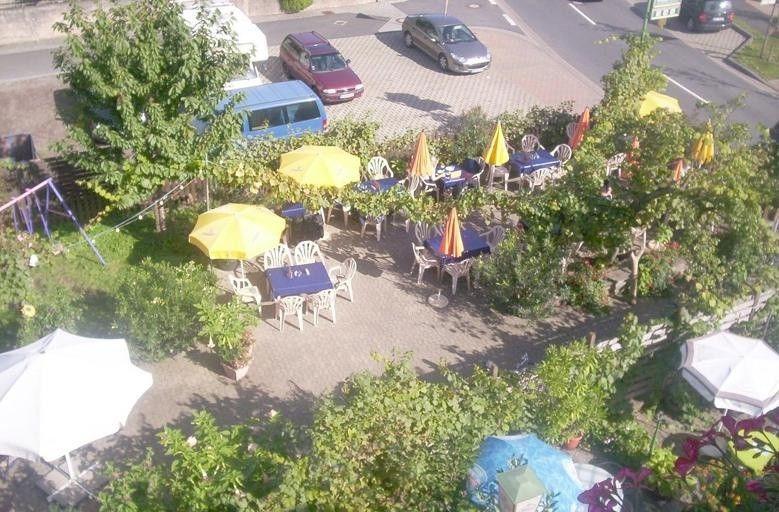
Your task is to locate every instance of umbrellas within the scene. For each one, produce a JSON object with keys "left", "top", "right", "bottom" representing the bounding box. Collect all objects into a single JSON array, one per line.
[
  {"left": 467, "top": 433, "right": 583, "bottom": 512},
  {"left": 678, "top": 330, "right": 779, "bottom": 431},
  {"left": 483, "top": 121, "right": 510, "bottom": 170},
  {"left": 189, "top": 203, "right": 286, "bottom": 290},
  {"left": 571, "top": 106, "right": 591, "bottom": 147},
  {"left": 410, "top": 129, "right": 433, "bottom": 182},
  {"left": 441, "top": 207, "right": 464, "bottom": 283},
  {"left": 280, "top": 145, "right": 361, "bottom": 188},
  {"left": 623, "top": 135, "right": 640, "bottom": 180},
  {"left": 637, "top": 90, "right": 682, "bottom": 123},
  {"left": 668, "top": 157, "right": 687, "bottom": 184},
  {"left": 0, "top": 328, "right": 152, "bottom": 481},
  {"left": 688, "top": 118, "right": 714, "bottom": 169}
]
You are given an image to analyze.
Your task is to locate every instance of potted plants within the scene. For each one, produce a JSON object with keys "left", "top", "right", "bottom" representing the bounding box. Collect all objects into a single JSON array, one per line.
[
  {"left": 553, "top": 381, "right": 596, "bottom": 449},
  {"left": 206, "top": 294, "right": 255, "bottom": 384}
]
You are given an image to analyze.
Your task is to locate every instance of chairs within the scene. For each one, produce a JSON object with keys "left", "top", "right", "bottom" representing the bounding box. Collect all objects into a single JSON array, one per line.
[
  {"left": 229, "top": 240, "right": 357, "bottom": 330},
  {"left": 404, "top": 206, "right": 520, "bottom": 294},
  {"left": 604, "top": 132, "right": 633, "bottom": 177},
  {"left": 420, "top": 156, "right": 486, "bottom": 202},
  {"left": 489, "top": 122, "right": 577, "bottom": 191},
  {"left": 281, "top": 156, "right": 419, "bottom": 240}
]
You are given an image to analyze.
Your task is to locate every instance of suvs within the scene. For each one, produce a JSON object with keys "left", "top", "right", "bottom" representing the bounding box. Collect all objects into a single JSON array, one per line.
[{"left": 279, "top": 31, "right": 366, "bottom": 105}]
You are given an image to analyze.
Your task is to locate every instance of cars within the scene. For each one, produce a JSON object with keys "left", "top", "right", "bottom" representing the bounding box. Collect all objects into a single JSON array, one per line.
[{"left": 402, "top": 13, "right": 492, "bottom": 74}]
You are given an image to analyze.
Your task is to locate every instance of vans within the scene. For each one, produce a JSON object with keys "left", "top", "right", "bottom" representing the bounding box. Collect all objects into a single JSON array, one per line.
[
  {"left": 187, "top": 79, "right": 329, "bottom": 153},
  {"left": 673, "top": 1, "right": 735, "bottom": 31}
]
